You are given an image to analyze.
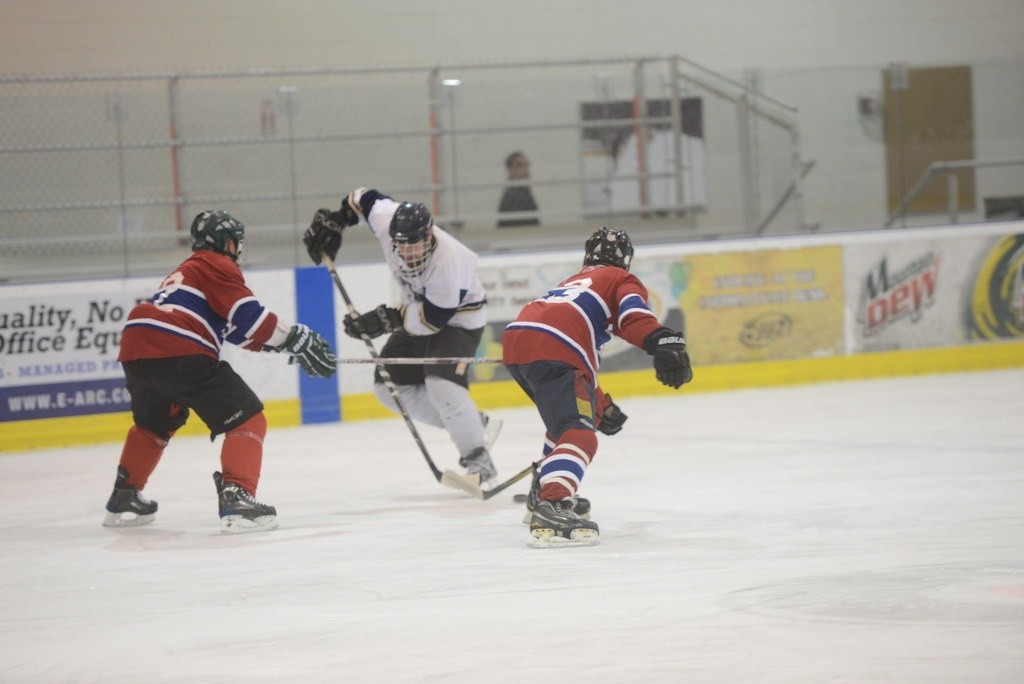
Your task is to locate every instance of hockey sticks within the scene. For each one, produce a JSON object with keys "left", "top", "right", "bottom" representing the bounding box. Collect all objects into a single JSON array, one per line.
[
  {"left": 318, "top": 249, "right": 484, "bottom": 489},
  {"left": 440, "top": 458, "right": 547, "bottom": 501},
  {"left": 286, "top": 352, "right": 504, "bottom": 368}
]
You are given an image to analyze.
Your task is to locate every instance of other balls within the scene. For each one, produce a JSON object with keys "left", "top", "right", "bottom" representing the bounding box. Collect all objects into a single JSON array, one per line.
[{"left": 513, "top": 494, "right": 527, "bottom": 503}]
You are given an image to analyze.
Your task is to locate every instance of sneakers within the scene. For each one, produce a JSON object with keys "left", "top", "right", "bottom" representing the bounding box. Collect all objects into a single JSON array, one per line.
[
  {"left": 521, "top": 462, "right": 591, "bottom": 525},
  {"left": 526, "top": 488, "right": 599, "bottom": 549},
  {"left": 213, "top": 471, "right": 279, "bottom": 534},
  {"left": 459, "top": 447, "right": 497, "bottom": 498},
  {"left": 476, "top": 409, "right": 503, "bottom": 454},
  {"left": 101, "top": 466, "right": 158, "bottom": 527}
]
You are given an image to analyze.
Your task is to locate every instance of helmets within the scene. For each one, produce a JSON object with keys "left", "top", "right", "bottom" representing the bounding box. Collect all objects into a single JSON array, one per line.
[
  {"left": 388, "top": 201, "right": 432, "bottom": 280},
  {"left": 190, "top": 209, "right": 246, "bottom": 268},
  {"left": 583, "top": 227, "right": 633, "bottom": 270}
]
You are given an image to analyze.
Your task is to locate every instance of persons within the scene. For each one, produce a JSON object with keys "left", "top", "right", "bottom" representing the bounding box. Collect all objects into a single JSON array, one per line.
[
  {"left": 497, "top": 152, "right": 539, "bottom": 226},
  {"left": 102, "top": 209, "right": 338, "bottom": 535},
  {"left": 302, "top": 187, "right": 503, "bottom": 492},
  {"left": 503, "top": 225, "right": 693, "bottom": 549}
]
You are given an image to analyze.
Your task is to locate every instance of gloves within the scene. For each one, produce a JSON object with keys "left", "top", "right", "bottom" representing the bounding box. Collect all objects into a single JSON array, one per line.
[
  {"left": 647, "top": 332, "right": 692, "bottom": 389},
  {"left": 304, "top": 212, "right": 344, "bottom": 265},
  {"left": 342, "top": 304, "right": 403, "bottom": 339},
  {"left": 596, "top": 404, "right": 629, "bottom": 436},
  {"left": 282, "top": 324, "right": 337, "bottom": 378}
]
